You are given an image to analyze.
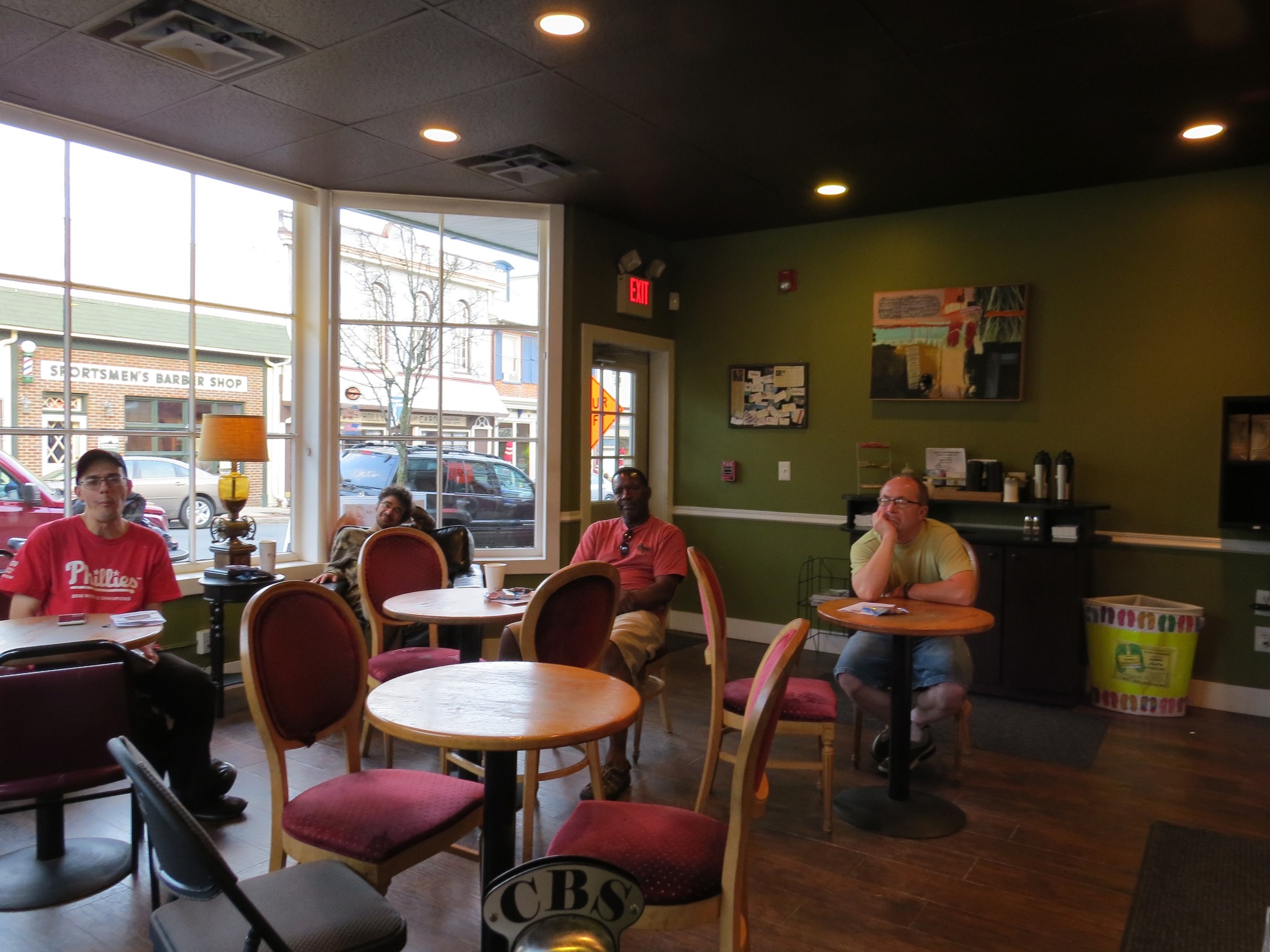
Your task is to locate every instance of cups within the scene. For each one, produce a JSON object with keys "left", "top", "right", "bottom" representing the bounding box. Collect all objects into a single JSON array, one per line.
[
  {"left": 258, "top": 540, "right": 277, "bottom": 573},
  {"left": 483, "top": 563, "right": 507, "bottom": 592}
]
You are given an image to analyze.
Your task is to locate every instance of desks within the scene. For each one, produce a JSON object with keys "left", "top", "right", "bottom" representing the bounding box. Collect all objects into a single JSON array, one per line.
[
  {"left": 0, "top": 608, "right": 165, "bottom": 913},
  {"left": 381, "top": 587, "right": 537, "bottom": 812},
  {"left": 363, "top": 660, "right": 643, "bottom": 952},
  {"left": 818, "top": 594, "right": 995, "bottom": 840}
]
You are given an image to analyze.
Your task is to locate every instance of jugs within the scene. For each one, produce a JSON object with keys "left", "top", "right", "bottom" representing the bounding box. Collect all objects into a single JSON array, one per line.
[
  {"left": 1055, "top": 451, "right": 1074, "bottom": 504},
  {"left": 1033, "top": 450, "right": 1051, "bottom": 503}
]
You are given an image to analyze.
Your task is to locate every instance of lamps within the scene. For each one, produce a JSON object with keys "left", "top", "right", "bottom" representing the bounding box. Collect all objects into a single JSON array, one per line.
[
  {"left": 616, "top": 248, "right": 665, "bottom": 319},
  {"left": 482, "top": 853, "right": 646, "bottom": 952},
  {"left": 20, "top": 339, "right": 37, "bottom": 383},
  {"left": 196, "top": 413, "right": 269, "bottom": 580}
]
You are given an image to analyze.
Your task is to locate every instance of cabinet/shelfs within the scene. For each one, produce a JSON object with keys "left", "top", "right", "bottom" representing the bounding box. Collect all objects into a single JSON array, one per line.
[
  {"left": 838, "top": 493, "right": 1113, "bottom": 711},
  {"left": 1217, "top": 395, "right": 1270, "bottom": 531}
]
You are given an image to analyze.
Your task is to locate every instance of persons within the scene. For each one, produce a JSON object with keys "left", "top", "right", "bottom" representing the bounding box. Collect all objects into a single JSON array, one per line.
[
  {"left": 309, "top": 485, "right": 453, "bottom": 647},
  {"left": 604, "top": 473, "right": 608, "bottom": 479},
  {"left": 498, "top": 467, "right": 687, "bottom": 800},
  {"left": 0, "top": 449, "right": 249, "bottom": 823},
  {"left": 834, "top": 475, "right": 975, "bottom": 774}
]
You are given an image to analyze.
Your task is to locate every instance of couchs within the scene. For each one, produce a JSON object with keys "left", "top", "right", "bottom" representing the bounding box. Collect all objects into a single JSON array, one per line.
[{"left": 316, "top": 524, "right": 485, "bottom": 666}]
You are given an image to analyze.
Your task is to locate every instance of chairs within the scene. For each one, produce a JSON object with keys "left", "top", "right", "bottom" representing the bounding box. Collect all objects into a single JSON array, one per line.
[
  {"left": 106, "top": 525, "right": 980, "bottom": 952},
  {"left": 0, "top": 640, "right": 147, "bottom": 912}
]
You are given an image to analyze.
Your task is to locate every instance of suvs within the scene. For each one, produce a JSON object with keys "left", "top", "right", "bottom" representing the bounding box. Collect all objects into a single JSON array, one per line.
[{"left": 337, "top": 444, "right": 536, "bottom": 547}]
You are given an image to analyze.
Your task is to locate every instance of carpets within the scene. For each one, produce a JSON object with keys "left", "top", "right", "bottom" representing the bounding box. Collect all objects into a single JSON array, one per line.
[
  {"left": 1119, "top": 821, "right": 1270, "bottom": 952},
  {"left": 814, "top": 669, "right": 1114, "bottom": 772}
]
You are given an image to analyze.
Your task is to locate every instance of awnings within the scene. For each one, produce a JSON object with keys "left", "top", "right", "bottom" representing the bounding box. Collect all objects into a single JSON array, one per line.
[{"left": 281, "top": 366, "right": 509, "bottom": 417}]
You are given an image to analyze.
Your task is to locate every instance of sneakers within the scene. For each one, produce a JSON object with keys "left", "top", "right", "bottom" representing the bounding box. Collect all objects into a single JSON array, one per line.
[
  {"left": 871, "top": 724, "right": 892, "bottom": 762},
  {"left": 580, "top": 761, "right": 631, "bottom": 802},
  {"left": 878, "top": 737, "right": 936, "bottom": 775}
]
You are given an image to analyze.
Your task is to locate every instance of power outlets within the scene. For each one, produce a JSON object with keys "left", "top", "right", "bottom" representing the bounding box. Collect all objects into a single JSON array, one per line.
[
  {"left": 777, "top": 461, "right": 791, "bottom": 482},
  {"left": 1253, "top": 626, "right": 1270, "bottom": 652}
]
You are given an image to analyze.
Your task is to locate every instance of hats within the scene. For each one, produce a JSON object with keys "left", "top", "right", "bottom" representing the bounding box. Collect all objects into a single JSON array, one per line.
[{"left": 76, "top": 449, "right": 128, "bottom": 477}]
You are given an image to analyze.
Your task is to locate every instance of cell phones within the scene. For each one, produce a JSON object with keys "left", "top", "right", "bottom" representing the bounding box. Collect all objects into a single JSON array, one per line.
[{"left": 57, "top": 613, "right": 87, "bottom": 625}]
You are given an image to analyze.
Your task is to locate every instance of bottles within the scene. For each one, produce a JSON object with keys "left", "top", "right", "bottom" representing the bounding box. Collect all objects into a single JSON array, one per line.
[
  {"left": 902, "top": 463, "right": 914, "bottom": 475},
  {"left": 1003, "top": 476, "right": 1018, "bottom": 503}
]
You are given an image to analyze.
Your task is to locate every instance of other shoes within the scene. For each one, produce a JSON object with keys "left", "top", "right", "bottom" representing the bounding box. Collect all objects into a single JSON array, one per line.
[
  {"left": 210, "top": 759, "right": 238, "bottom": 795},
  {"left": 188, "top": 796, "right": 247, "bottom": 823}
]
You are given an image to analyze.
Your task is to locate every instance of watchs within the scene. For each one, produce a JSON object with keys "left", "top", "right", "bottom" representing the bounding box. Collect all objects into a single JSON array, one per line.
[{"left": 903, "top": 582, "right": 915, "bottom": 599}]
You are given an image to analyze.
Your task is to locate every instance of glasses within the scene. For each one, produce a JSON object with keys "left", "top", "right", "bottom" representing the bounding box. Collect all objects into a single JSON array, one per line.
[
  {"left": 620, "top": 530, "right": 632, "bottom": 557},
  {"left": 876, "top": 497, "right": 922, "bottom": 507},
  {"left": 380, "top": 501, "right": 403, "bottom": 516},
  {"left": 78, "top": 477, "right": 128, "bottom": 490}
]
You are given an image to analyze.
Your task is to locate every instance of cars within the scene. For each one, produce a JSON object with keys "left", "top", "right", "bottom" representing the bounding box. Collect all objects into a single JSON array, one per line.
[
  {"left": 38, "top": 455, "right": 223, "bottom": 531},
  {"left": 0, "top": 451, "right": 170, "bottom": 595}
]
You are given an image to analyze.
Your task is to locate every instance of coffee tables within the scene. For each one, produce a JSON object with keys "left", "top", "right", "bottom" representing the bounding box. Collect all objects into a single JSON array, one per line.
[{"left": 196, "top": 572, "right": 286, "bottom": 720}]
